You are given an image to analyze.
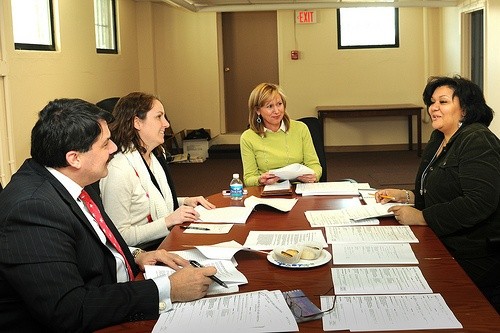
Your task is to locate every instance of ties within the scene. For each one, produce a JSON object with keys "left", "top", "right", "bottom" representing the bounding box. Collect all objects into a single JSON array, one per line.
[{"left": 79, "top": 189, "right": 136, "bottom": 282}]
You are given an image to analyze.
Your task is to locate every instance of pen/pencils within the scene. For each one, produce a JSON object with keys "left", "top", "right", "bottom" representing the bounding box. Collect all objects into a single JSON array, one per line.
[
  {"left": 189, "top": 260, "right": 229, "bottom": 288},
  {"left": 368, "top": 193, "right": 396, "bottom": 200},
  {"left": 179, "top": 225, "right": 210, "bottom": 230}
]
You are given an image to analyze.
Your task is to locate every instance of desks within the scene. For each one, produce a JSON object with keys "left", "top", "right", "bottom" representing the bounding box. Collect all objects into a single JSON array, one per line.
[
  {"left": 93, "top": 186, "right": 500, "bottom": 333},
  {"left": 316, "top": 103, "right": 424, "bottom": 158}
]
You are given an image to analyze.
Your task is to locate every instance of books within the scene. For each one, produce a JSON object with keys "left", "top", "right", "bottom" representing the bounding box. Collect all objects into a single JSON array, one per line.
[{"left": 260, "top": 182, "right": 292, "bottom": 195}]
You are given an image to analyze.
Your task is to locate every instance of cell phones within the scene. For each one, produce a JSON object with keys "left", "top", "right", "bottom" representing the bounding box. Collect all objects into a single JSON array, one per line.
[{"left": 222, "top": 190, "right": 248, "bottom": 195}]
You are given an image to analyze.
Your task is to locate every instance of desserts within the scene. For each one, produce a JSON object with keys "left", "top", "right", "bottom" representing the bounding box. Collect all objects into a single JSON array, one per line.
[{"left": 280, "top": 244, "right": 319, "bottom": 262}]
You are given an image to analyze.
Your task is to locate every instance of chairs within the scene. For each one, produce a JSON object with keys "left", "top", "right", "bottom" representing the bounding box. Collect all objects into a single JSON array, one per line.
[{"left": 247, "top": 117, "right": 327, "bottom": 182}]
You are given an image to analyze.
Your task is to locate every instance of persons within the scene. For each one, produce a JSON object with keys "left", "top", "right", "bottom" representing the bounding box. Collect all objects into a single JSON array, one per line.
[
  {"left": 0, "top": 97, "right": 218, "bottom": 333},
  {"left": 239, "top": 83, "right": 322, "bottom": 186},
  {"left": 99, "top": 93, "right": 216, "bottom": 251},
  {"left": 375, "top": 74, "right": 500, "bottom": 315}
]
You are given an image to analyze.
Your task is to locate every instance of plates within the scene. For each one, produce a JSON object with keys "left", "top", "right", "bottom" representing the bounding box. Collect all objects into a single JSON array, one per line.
[{"left": 266, "top": 248, "right": 332, "bottom": 268}]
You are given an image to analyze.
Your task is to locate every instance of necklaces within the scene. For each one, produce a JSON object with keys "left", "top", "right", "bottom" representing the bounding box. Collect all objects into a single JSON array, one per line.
[{"left": 420, "top": 122, "right": 461, "bottom": 196}]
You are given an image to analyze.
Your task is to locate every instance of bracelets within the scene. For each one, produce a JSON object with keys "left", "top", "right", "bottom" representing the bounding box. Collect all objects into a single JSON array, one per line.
[
  {"left": 403, "top": 189, "right": 410, "bottom": 204},
  {"left": 133, "top": 249, "right": 146, "bottom": 258}
]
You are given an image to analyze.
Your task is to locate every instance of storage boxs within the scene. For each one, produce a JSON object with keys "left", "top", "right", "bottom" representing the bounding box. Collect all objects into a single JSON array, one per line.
[{"left": 181, "top": 128, "right": 211, "bottom": 159}]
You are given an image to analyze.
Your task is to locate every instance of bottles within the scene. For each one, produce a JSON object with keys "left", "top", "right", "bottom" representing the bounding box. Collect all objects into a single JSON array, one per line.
[{"left": 230, "top": 173, "right": 243, "bottom": 201}]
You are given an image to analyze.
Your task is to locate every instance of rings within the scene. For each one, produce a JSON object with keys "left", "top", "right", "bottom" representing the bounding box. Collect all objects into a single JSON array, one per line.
[
  {"left": 309, "top": 181, "right": 311, "bottom": 183},
  {"left": 395, "top": 216, "right": 398, "bottom": 220}
]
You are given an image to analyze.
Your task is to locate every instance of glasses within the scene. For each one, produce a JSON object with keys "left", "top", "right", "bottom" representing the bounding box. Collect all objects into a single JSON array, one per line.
[{"left": 285, "top": 287, "right": 337, "bottom": 320}]
